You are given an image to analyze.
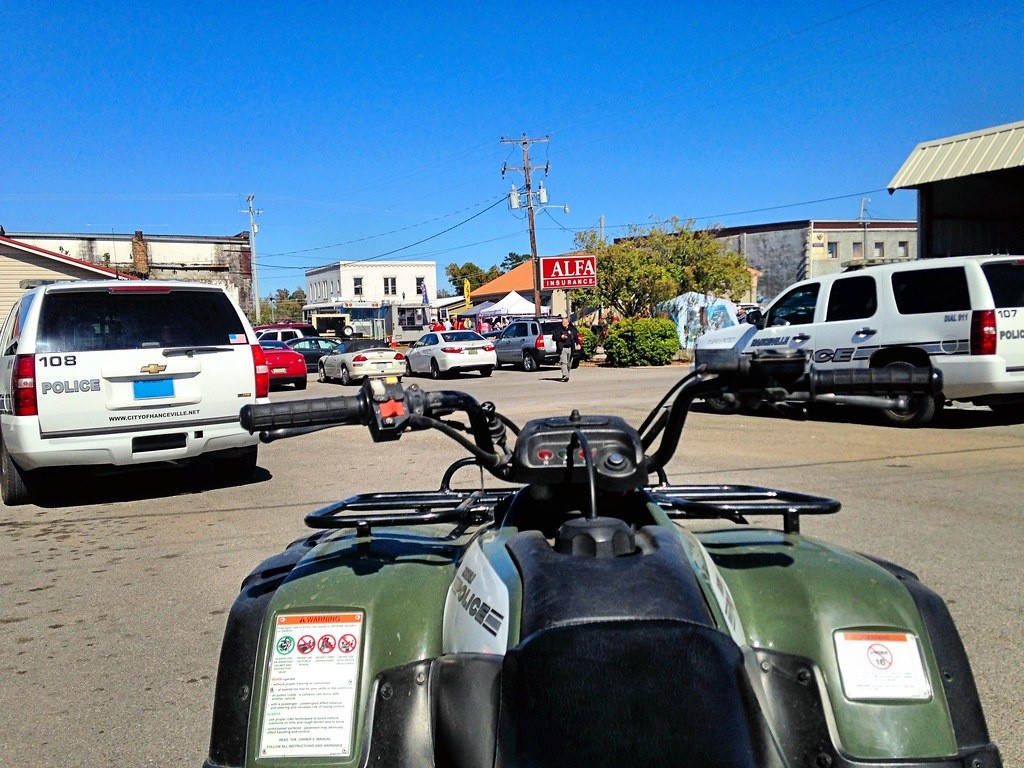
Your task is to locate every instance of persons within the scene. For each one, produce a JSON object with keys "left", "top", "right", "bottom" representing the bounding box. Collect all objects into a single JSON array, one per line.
[
  {"left": 428, "top": 318, "right": 510, "bottom": 334},
  {"left": 552, "top": 318, "right": 581, "bottom": 382},
  {"left": 737, "top": 309, "right": 747, "bottom": 324}
]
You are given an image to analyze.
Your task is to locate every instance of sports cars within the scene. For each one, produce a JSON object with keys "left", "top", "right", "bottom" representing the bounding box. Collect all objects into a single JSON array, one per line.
[{"left": 318, "top": 339, "right": 407, "bottom": 385}]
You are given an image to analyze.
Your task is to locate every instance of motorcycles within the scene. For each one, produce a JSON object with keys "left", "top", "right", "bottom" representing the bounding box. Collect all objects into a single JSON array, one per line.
[{"left": 204, "top": 348, "right": 1001, "bottom": 768}]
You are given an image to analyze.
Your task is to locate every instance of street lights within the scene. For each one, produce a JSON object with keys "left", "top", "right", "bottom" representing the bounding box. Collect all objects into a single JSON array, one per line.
[{"left": 529, "top": 202, "right": 570, "bottom": 315}]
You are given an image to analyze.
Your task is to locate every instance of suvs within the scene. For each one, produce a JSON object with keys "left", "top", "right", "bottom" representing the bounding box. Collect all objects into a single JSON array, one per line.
[
  {"left": 692, "top": 252, "right": 1023, "bottom": 427},
  {"left": 491, "top": 316, "right": 584, "bottom": 372},
  {"left": 0, "top": 277, "right": 273, "bottom": 508}
]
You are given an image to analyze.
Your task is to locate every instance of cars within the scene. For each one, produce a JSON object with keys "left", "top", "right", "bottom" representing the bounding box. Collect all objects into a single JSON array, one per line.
[
  {"left": 253, "top": 328, "right": 340, "bottom": 390},
  {"left": 404, "top": 329, "right": 497, "bottom": 379}
]
